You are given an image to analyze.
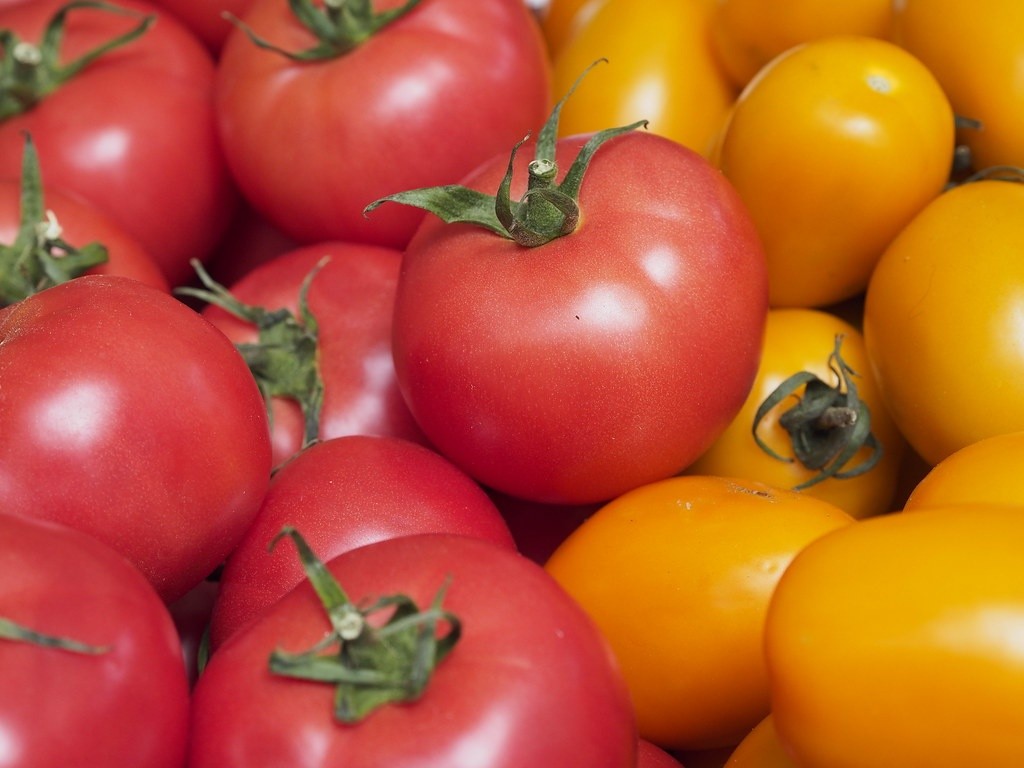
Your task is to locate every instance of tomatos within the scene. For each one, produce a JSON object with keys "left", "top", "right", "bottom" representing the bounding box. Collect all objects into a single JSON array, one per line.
[{"left": 0, "top": 0, "right": 1024, "bottom": 768}]
